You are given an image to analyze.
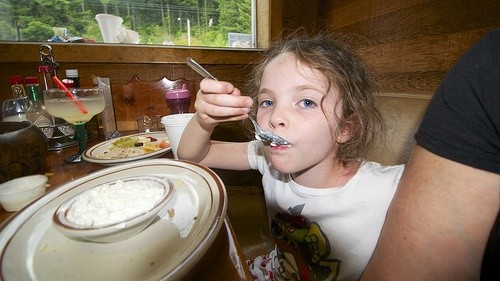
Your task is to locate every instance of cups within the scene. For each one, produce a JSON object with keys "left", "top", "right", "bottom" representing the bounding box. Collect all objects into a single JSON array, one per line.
[
  {"left": 95, "top": 13, "right": 124, "bottom": 43},
  {"left": 2, "top": 97, "right": 33, "bottom": 123},
  {"left": 160, "top": 113, "right": 195, "bottom": 159}
]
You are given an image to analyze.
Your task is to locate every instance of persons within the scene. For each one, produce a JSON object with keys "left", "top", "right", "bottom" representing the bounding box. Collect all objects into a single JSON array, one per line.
[
  {"left": 359, "top": 29, "right": 500, "bottom": 281},
  {"left": 177, "top": 21, "right": 406, "bottom": 281}
]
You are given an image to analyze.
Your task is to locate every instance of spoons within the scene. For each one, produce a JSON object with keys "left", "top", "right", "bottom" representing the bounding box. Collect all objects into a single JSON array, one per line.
[{"left": 186, "top": 57, "right": 292, "bottom": 147}]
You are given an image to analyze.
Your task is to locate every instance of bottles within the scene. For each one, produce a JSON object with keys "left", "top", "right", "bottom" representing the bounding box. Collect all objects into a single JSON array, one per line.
[
  {"left": 66, "top": 70, "right": 99, "bottom": 142},
  {"left": 25, "top": 77, "right": 54, "bottom": 140},
  {"left": 8, "top": 75, "right": 27, "bottom": 98},
  {"left": 52, "top": 78, "right": 76, "bottom": 143},
  {"left": 38, "top": 66, "right": 54, "bottom": 105},
  {"left": 91, "top": 76, "right": 105, "bottom": 133},
  {"left": 165, "top": 84, "right": 191, "bottom": 115}
]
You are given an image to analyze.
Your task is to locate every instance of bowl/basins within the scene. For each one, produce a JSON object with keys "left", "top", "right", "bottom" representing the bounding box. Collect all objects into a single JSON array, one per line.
[
  {"left": 0, "top": 121, "right": 49, "bottom": 184},
  {"left": 0, "top": 175, "right": 48, "bottom": 211},
  {"left": 52, "top": 175, "right": 174, "bottom": 243},
  {"left": 228, "top": 33, "right": 253, "bottom": 48}
]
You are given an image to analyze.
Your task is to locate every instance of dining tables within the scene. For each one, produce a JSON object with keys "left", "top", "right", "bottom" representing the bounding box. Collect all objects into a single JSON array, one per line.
[{"left": 0, "top": 128, "right": 253, "bottom": 281}]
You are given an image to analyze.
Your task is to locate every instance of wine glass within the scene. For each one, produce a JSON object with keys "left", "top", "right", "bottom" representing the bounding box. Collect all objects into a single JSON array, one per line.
[{"left": 43, "top": 89, "right": 105, "bottom": 163}]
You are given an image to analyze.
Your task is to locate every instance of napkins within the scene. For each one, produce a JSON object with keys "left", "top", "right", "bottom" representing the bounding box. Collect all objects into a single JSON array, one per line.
[{"left": 117, "top": 27, "right": 140, "bottom": 44}]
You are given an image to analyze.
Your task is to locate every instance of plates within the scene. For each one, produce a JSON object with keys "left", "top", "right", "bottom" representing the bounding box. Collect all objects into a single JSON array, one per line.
[
  {"left": 0, "top": 158, "right": 228, "bottom": 281},
  {"left": 82, "top": 131, "right": 171, "bottom": 167}
]
